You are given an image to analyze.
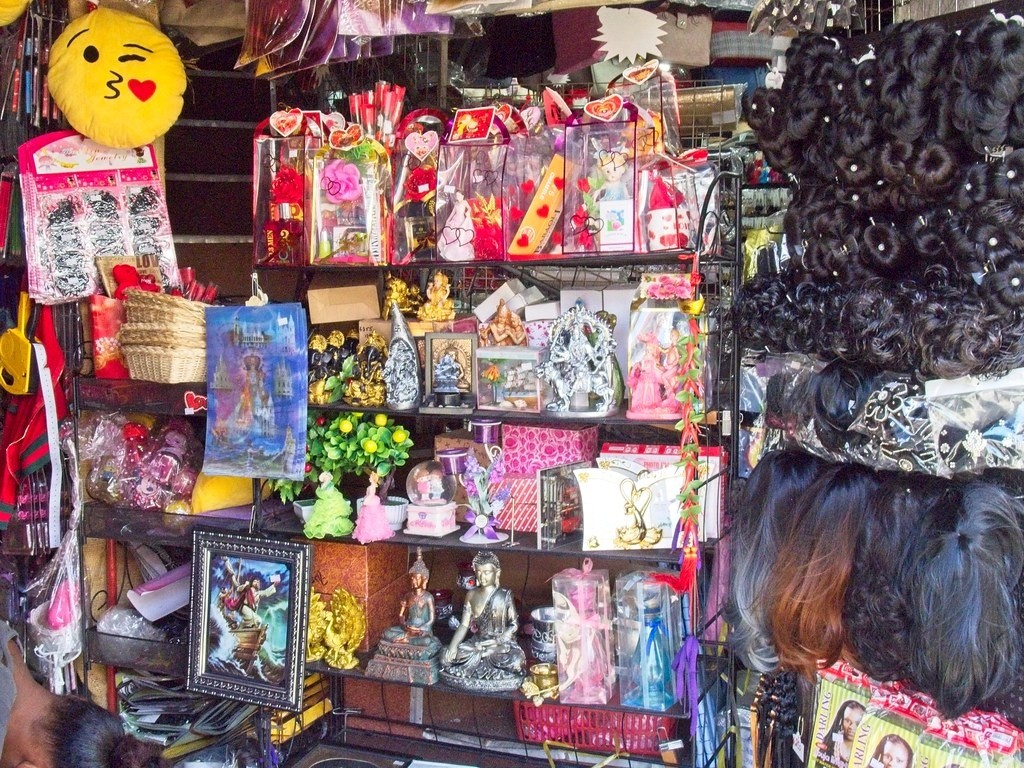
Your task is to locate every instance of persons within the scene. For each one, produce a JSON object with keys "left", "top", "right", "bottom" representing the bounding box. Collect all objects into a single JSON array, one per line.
[
  {"left": 813, "top": 700, "right": 867, "bottom": 768},
  {"left": 867, "top": 734, "right": 913, "bottom": 767},
  {"left": 312, "top": 271, "right": 627, "bottom": 416},
  {"left": 440, "top": 550, "right": 529, "bottom": 675},
  {"left": 380, "top": 547, "right": 442, "bottom": 657},
  {"left": 230, "top": 571, "right": 280, "bottom": 628},
  {"left": 217, "top": 585, "right": 236, "bottom": 629},
  {"left": 0, "top": 619, "right": 170, "bottom": 768}
]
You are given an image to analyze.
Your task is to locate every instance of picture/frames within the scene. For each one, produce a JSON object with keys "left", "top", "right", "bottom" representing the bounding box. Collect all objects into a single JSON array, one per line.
[{"left": 184, "top": 525, "right": 314, "bottom": 711}]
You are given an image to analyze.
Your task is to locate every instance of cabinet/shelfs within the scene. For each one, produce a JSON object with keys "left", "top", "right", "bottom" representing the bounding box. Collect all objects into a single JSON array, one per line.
[{"left": 67, "top": 172, "right": 749, "bottom": 764}]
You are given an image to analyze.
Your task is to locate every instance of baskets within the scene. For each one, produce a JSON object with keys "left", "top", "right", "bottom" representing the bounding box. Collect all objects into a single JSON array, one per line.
[{"left": 122, "top": 288, "right": 223, "bottom": 384}]
